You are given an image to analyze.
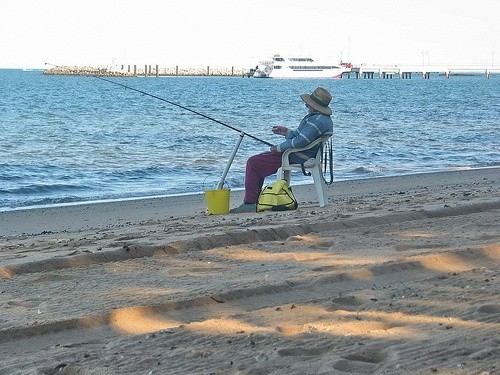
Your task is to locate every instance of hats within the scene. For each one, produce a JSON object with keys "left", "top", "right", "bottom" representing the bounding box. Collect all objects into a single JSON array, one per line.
[{"left": 300, "top": 87, "right": 332, "bottom": 115}]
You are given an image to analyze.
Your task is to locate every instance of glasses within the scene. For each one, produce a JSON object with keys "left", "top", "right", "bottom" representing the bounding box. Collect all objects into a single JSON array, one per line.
[{"left": 305, "top": 104, "right": 309, "bottom": 108}]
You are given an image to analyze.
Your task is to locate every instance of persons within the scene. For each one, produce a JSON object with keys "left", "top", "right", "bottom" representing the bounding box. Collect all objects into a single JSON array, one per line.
[{"left": 230, "top": 86, "right": 333, "bottom": 213}]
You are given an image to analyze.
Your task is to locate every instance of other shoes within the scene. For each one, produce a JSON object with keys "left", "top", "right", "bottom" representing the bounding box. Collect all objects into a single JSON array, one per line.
[{"left": 230, "top": 203, "right": 257, "bottom": 213}]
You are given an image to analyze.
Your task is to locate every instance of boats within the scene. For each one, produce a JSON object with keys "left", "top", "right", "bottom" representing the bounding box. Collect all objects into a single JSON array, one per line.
[{"left": 252, "top": 52, "right": 352, "bottom": 79}]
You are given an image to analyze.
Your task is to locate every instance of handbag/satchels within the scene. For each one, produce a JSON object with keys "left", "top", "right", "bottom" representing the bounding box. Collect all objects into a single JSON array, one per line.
[{"left": 256, "top": 179, "right": 297, "bottom": 212}]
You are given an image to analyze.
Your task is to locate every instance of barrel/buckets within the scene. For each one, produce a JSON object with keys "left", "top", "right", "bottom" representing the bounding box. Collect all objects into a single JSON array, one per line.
[{"left": 203, "top": 175, "right": 231, "bottom": 215}]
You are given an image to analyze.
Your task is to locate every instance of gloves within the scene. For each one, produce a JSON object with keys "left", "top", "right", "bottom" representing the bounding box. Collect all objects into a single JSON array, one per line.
[
  {"left": 270, "top": 146, "right": 277, "bottom": 152},
  {"left": 272, "top": 126, "right": 287, "bottom": 135}
]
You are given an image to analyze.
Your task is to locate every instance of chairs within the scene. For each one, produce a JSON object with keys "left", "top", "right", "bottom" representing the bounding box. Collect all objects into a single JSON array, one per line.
[{"left": 277, "top": 135, "right": 329, "bottom": 207}]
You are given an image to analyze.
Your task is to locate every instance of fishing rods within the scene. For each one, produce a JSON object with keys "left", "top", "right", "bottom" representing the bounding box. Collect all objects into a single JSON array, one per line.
[{"left": 46, "top": 61, "right": 277, "bottom": 149}]
